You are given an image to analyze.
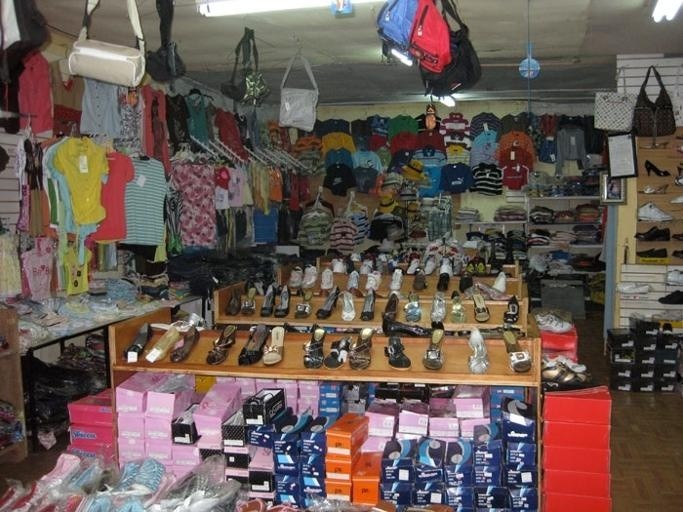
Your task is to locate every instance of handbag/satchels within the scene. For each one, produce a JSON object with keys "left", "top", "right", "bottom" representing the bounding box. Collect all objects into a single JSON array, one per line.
[
  {"left": 219, "top": 24, "right": 270, "bottom": 110},
  {"left": 0, "top": 1, "right": 46, "bottom": 58},
  {"left": 277, "top": 52, "right": 321, "bottom": 131},
  {"left": 407, "top": 0, "right": 451, "bottom": 73},
  {"left": 419, "top": 0, "right": 482, "bottom": 97},
  {"left": 376, "top": 0, "right": 418, "bottom": 65},
  {"left": 593, "top": 66, "right": 635, "bottom": 133},
  {"left": 146, "top": 1, "right": 187, "bottom": 83},
  {"left": 632, "top": 65, "right": 676, "bottom": 138},
  {"left": 68, "top": 0, "right": 146, "bottom": 88}
]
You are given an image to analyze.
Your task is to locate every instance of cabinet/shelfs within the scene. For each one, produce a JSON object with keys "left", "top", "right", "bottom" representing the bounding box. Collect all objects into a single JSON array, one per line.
[
  {"left": 278, "top": 263, "right": 525, "bottom": 297},
  {"left": 213, "top": 285, "right": 529, "bottom": 327},
  {"left": 316, "top": 255, "right": 521, "bottom": 276},
  {"left": 21, "top": 249, "right": 281, "bottom": 452},
  {"left": 108, "top": 307, "right": 541, "bottom": 512},
  {"left": 448, "top": 207, "right": 527, "bottom": 263},
  {"left": 524, "top": 183, "right": 605, "bottom": 306}
]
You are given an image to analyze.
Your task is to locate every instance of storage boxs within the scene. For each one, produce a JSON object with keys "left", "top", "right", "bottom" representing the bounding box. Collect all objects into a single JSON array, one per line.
[
  {"left": 541, "top": 386, "right": 612, "bottom": 512},
  {"left": 605, "top": 316, "right": 682, "bottom": 394},
  {"left": 62, "top": 390, "right": 119, "bottom": 464}
]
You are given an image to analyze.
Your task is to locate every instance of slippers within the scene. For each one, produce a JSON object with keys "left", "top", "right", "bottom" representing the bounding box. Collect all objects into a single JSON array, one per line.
[{"left": 322, "top": 339, "right": 348, "bottom": 370}]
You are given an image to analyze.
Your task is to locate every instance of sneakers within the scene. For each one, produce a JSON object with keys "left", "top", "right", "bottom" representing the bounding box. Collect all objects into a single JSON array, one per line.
[
  {"left": 466, "top": 261, "right": 475, "bottom": 273},
  {"left": 541, "top": 359, "right": 586, "bottom": 385},
  {"left": 672, "top": 233, "right": 683, "bottom": 240},
  {"left": 387, "top": 258, "right": 404, "bottom": 272},
  {"left": 672, "top": 249, "right": 683, "bottom": 258},
  {"left": 633, "top": 226, "right": 671, "bottom": 241},
  {"left": 288, "top": 266, "right": 302, "bottom": 288},
  {"left": 459, "top": 270, "right": 473, "bottom": 293},
  {"left": 424, "top": 255, "right": 436, "bottom": 275},
  {"left": 390, "top": 269, "right": 403, "bottom": 290},
  {"left": 662, "top": 322, "right": 672, "bottom": 334},
  {"left": 536, "top": 315, "right": 572, "bottom": 333},
  {"left": 440, "top": 257, "right": 453, "bottom": 276},
  {"left": 636, "top": 247, "right": 667, "bottom": 258},
  {"left": 492, "top": 272, "right": 506, "bottom": 292},
  {"left": 476, "top": 258, "right": 486, "bottom": 275},
  {"left": 637, "top": 202, "right": 673, "bottom": 221},
  {"left": 365, "top": 270, "right": 382, "bottom": 291},
  {"left": 302, "top": 266, "right": 318, "bottom": 289},
  {"left": 333, "top": 261, "right": 347, "bottom": 273},
  {"left": 321, "top": 269, "right": 333, "bottom": 289},
  {"left": 407, "top": 259, "right": 419, "bottom": 275},
  {"left": 436, "top": 272, "right": 449, "bottom": 292},
  {"left": 543, "top": 353, "right": 586, "bottom": 373},
  {"left": 351, "top": 252, "right": 361, "bottom": 262},
  {"left": 413, "top": 269, "right": 425, "bottom": 290},
  {"left": 347, "top": 270, "right": 359, "bottom": 290},
  {"left": 359, "top": 259, "right": 373, "bottom": 274},
  {"left": 665, "top": 269, "right": 683, "bottom": 286},
  {"left": 617, "top": 283, "right": 648, "bottom": 294},
  {"left": 658, "top": 290, "right": 683, "bottom": 305},
  {"left": 669, "top": 195, "right": 683, "bottom": 203}
]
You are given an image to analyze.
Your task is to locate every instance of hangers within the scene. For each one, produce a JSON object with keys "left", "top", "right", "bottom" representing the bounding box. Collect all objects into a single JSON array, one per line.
[
  {"left": 111, "top": 137, "right": 143, "bottom": 155},
  {"left": 241, "top": 144, "right": 316, "bottom": 178},
  {"left": 82, "top": 133, "right": 110, "bottom": 146},
  {"left": 165, "top": 86, "right": 286, "bottom": 132},
  {"left": 286, "top": 183, "right": 449, "bottom": 226},
  {"left": 50, "top": 44, "right": 73, "bottom": 78},
  {"left": 208, "top": 137, "right": 232, "bottom": 162},
  {"left": 169, "top": 141, "right": 190, "bottom": 160},
  {"left": 189, "top": 134, "right": 216, "bottom": 166},
  {"left": 216, "top": 134, "right": 248, "bottom": 169},
  {"left": 54, "top": 119, "right": 78, "bottom": 137}
]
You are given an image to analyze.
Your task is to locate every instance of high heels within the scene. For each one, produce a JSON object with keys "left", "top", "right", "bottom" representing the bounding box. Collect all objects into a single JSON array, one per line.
[
  {"left": 403, "top": 293, "right": 421, "bottom": 322},
  {"left": 645, "top": 160, "right": 670, "bottom": 176},
  {"left": 122, "top": 322, "right": 152, "bottom": 360},
  {"left": 237, "top": 324, "right": 269, "bottom": 366},
  {"left": 423, "top": 320, "right": 447, "bottom": 370},
  {"left": 315, "top": 293, "right": 338, "bottom": 318},
  {"left": 450, "top": 296, "right": 467, "bottom": 323},
  {"left": 224, "top": 288, "right": 241, "bottom": 316},
  {"left": 302, "top": 328, "right": 325, "bottom": 370},
  {"left": 341, "top": 292, "right": 357, "bottom": 321},
  {"left": 676, "top": 161, "right": 683, "bottom": 175},
  {"left": 637, "top": 183, "right": 669, "bottom": 194},
  {"left": 471, "top": 294, "right": 490, "bottom": 322},
  {"left": 241, "top": 288, "right": 257, "bottom": 316},
  {"left": 385, "top": 334, "right": 411, "bottom": 371},
  {"left": 262, "top": 327, "right": 285, "bottom": 366},
  {"left": 205, "top": 325, "right": 236, "bottom": 365},
  {"left": 383, "top": 293, "right": 399, "bottom": 320},
  {"left": 273, "top": 284, "right": 291, "bottom": 317},
  {"left": 294, "top": 291, "right": 313, "bottom": 319},
  {"left": 430, "top": 291, "right": 448, "bottom": 323},
  {"left": 468, "top": 327, "right": 488, "bottom": 374},
  {"left": 360, "top": 288, "right": 376, "bottom": 320},
  {"left": 348, "top": 326, "right": 373, "bottom": 371},
  {"left": 260, "top": 285, "right": 277, "bottom": 317},
  {"left": 502, "top": 296, "right": 519, "bottom": 324}
]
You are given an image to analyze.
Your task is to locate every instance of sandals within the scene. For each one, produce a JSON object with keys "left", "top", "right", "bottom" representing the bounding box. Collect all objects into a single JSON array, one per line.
[
  {"left": 169, "top": 326, "right": 200, "bottom": 363},
  {"left": 150, "top": 328, "right": 179, "bottom": 362},
  {"left": 382, "top": 318, "right": 429, "bottom": 337},
  {"left": 0, "top": 452, "right": 242, "bottom": 512}
]
